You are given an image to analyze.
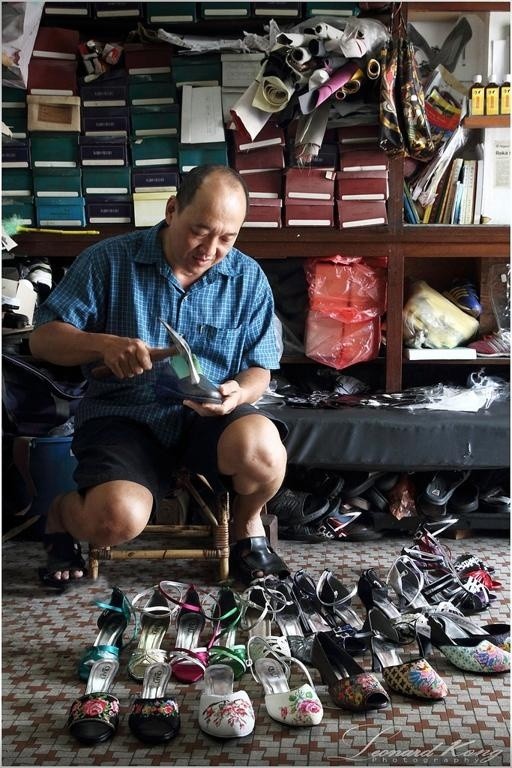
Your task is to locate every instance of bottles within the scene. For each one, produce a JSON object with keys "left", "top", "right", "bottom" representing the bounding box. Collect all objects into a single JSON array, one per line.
[
  {"left": 500, "top": 73, "right": 510, "bottom": 115},
  {"left": 469, "top": 73, "right": 485, "bottom": 116},
  {"left": 485, "top": 74, "right": 499, "bottom": 116}
]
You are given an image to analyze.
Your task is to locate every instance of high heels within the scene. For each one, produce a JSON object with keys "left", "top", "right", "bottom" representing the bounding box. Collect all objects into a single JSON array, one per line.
[{"left": 66, "top": 518, "right": 512, "bottom": 744}]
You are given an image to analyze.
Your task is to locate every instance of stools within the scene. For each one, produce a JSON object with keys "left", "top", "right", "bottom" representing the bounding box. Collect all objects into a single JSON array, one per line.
[{"left": 83, "top": 458, "right": 233, "bottom": 583}]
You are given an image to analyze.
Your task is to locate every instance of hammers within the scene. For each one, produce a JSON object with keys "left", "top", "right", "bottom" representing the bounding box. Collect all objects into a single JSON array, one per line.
[{"left": 91, "top": 318, "right": 200, "bottom": 386}]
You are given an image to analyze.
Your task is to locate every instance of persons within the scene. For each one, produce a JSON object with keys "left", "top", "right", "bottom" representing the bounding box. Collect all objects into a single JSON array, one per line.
[{"left": 29, "top": 165, "right": 289, "bottom": 590}]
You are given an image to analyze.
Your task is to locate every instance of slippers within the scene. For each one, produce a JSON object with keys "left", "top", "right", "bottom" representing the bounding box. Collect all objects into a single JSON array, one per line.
[{"left": 264, "top": 471, "right": 510, "bottom": 543}]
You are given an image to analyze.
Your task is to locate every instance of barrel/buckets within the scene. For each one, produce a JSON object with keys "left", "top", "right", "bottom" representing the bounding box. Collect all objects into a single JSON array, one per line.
[{"left": 26, "top": 437, "right": 79, "bottom": 523}]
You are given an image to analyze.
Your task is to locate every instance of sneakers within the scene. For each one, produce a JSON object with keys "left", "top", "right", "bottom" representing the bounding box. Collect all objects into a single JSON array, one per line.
[
  {"left": 442, "top": 276, "right": 481, "bottom": 317},
  {"left": 468, "top": 329, "right": 510, "bottom": 357},
  {"left": 4, "top": 497, "right": 38, "bottom": 540}
]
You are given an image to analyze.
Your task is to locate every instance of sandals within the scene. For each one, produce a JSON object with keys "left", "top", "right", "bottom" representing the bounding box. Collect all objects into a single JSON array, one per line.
[
  {"left": 230, "top": 536, "right": 291, "bottom": 585},
  {"left": 41, "top": 529, "right": 89, "bottom": 584}
]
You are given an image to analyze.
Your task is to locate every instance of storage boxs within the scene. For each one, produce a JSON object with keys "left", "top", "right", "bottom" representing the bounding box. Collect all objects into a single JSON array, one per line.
[{"left": 1, "top": 0, "right": 388, "bottom": 229}]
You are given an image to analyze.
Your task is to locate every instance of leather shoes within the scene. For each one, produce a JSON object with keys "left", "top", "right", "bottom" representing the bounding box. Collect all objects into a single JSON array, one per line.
[{"left": 155, "top": 361, "right": 224, "bottom": 404}]
